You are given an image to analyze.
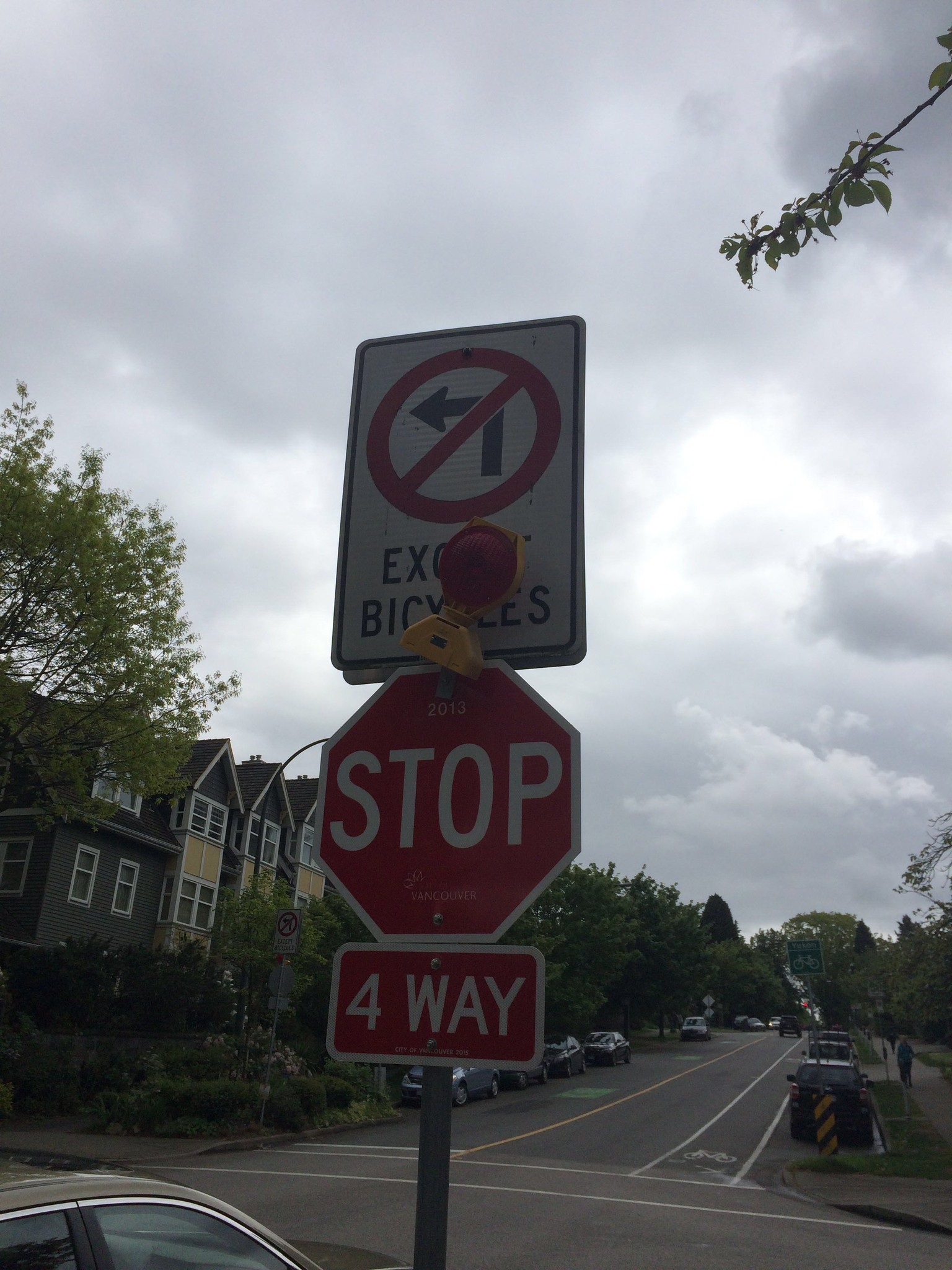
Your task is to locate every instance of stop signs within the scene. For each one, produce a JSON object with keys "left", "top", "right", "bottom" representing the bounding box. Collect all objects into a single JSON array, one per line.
[{"left": 309, "top": 655, "right": 583, "bottom": 945}]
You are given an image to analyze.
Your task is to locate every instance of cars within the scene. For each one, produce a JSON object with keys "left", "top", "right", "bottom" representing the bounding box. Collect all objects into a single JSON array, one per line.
[
  {"left": 740, "top": 1017, "right": 767, "bottom": 1032},
  {"left": 680, "top": 1017, "right": 711, "bottom": 1041},
  {"left": 400, "top": 1064, "right": 500, "bottom": 1108},
  {"left": 498, "top": 1034, "right": 588, "bottom": 1091},
  {"left": 733, "top": 1015, "right": 749, "bottom": 1030},
  {"left": 778, "top": 1015, "right": 803, "bottom": 1037},
  {"left": 580, "top": 1032, "right": 632, "bottom": 1066},
  {"left": 768, "top": 1016, "right": 782, "bottom": 1030},
  {"left": 1, "top": 1145, "right": 410, "bottom": 1270},
  {"left": 786, "top": 1025, "right": 875, "bottom": 1148}
]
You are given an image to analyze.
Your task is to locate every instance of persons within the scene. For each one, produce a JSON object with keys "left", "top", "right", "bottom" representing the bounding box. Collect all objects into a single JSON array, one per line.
[{"left": 897, "top": 1037, "right": 914, "bottom": 1089}]
